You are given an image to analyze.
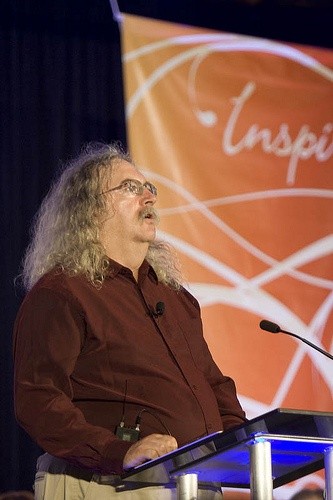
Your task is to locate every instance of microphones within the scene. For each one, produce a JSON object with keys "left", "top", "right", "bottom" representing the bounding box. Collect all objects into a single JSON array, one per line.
[
  {"left": 258, "top": 319, "right": 333, "bottom": 360},
  {"left": 149, "top": 300, "right": 166, "bottom": 317}
]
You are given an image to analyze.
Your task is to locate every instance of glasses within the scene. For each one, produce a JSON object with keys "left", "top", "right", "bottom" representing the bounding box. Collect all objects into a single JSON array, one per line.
[{"left": 97, "top": 179, "right": 157, "bottom": 198}]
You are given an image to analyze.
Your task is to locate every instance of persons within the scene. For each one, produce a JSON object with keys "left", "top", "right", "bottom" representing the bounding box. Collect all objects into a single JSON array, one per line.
[{"left": 11, "top": 147, "right": 248, "bottom": 500}]
